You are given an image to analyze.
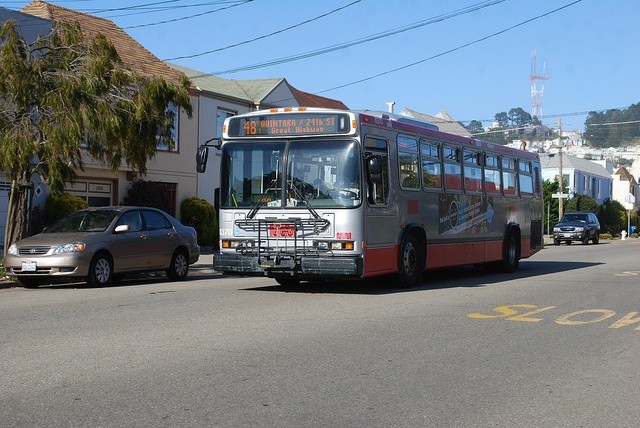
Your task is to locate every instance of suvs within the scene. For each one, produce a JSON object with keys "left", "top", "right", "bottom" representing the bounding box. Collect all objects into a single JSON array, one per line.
[{"left": 553, "top": 212, "right": 601, "bottom": 245}]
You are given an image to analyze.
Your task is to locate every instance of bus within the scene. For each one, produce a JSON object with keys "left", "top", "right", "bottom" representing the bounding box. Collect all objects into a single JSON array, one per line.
[{"left": 196, "top": 106, "right": 544, "bottom": 289}]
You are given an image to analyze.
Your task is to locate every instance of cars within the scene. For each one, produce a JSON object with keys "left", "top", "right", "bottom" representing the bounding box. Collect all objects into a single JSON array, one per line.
[{"left": 4, "top": 205, "right": 200, "bottom": 288}]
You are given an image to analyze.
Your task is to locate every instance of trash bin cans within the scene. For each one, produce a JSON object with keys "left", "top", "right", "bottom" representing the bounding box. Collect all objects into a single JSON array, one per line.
[{"left": 631, "top": 226, "right": 637, "bottom": 235}]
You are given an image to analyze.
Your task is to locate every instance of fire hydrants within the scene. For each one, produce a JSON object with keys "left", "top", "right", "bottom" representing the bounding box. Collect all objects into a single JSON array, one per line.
[{"left": 621, "top": 230, "right": 627, "bottom": 240}]
